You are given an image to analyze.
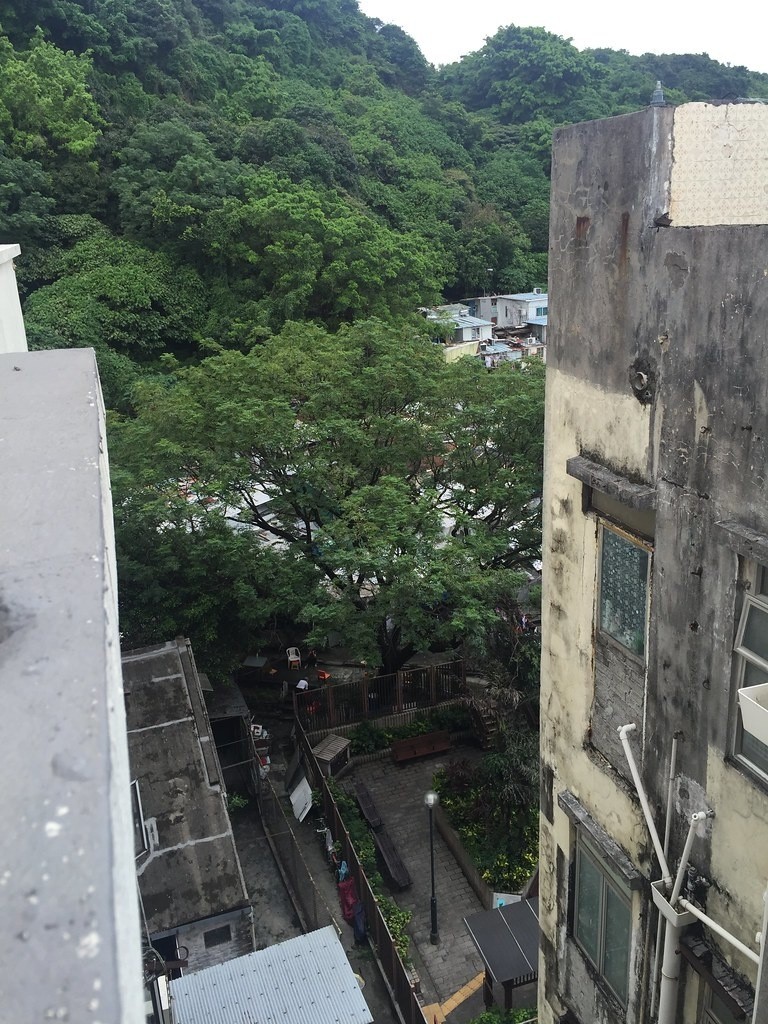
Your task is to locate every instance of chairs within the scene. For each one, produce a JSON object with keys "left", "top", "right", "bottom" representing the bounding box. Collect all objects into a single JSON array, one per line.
[
  {"left": 286, "top": 647, "right": 301, "bottom": 669},
  {"left": 318, "top": 670, "right": 331, "bottom": 681}
]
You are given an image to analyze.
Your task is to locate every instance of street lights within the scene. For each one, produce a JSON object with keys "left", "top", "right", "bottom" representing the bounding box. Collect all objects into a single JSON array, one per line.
[{"left": 424, "top": 790, "right": 443, "bottom": 946}]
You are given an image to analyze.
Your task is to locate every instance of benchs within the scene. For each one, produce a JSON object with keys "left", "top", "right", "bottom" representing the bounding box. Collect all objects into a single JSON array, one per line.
[
  {"left": 350, "top": 781, "right": 386, "bottom": 828},
  {"left": 370, "top": 828, "right": 414, "bottom": 892},
  {"left": 391, "top": 731, "right": 453, "bottom": 767}
]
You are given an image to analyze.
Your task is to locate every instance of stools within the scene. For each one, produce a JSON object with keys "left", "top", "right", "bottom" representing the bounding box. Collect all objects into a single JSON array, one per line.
[{"left": 291, "top": 661, "right": 299, "bottom": 670}]
